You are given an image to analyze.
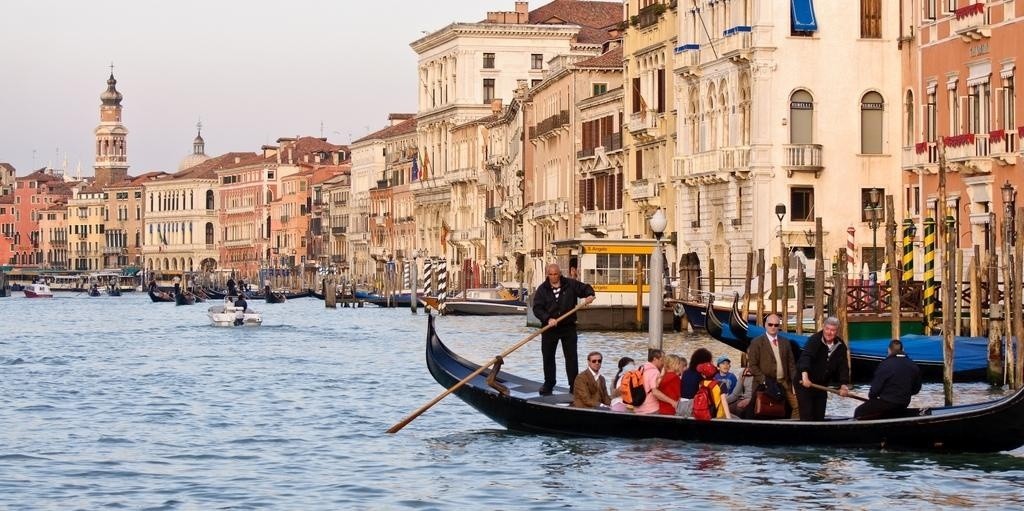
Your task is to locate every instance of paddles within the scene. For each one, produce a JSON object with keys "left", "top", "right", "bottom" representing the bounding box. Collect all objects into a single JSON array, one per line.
[{"left": 387, "top": 300, "right": 588, "bottom": 434}]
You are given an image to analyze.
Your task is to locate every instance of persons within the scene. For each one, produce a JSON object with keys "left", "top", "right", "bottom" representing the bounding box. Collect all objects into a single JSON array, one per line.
[
  {"left": 794, "top": 318, "right": 849, "bottom": 420},
  {"left": 188, "top": 277, "right": 194, "bottom": 293},
  {"left": 224, "top": 297, "right": 233, "bottom": 314},
  {"left": 747, "top": 312, "right": 797, "bottom": 417},
  {"left": 75, "top": 278, "right": 83, "bottom": 288},
  {"left": 171, "top": 275, "right": 181, "bottom": 295},
  {"left": 227, "top": 277, "right": 236, "bottom": 294},
  {"left": 533, "top": 264, "right": 595, "bottom": 396},
  {"left": 31, "top": 277, "right": 50, "bottom": 286},
  {"left": 149, "top": 278, "right": 156, "bottom": 290},
  {"left": 105, "top": 277, "right": 119, "bottom": 290},
  {"left": 611, "top": 349, "right": 753, "bottom": 421},
  {"left": 238, "top": 278, "right": 244, "bottom": 292},
  {"left": 234, "top": 295, "right": 247, "bottom": 312},
  {"left": 264, "top": 277, "right": 272, "bottom": 294},
  {"left": 243, "top": 278, "right": 249, "bottom": 292},
  {"left": 571, "top": 352, "right": 611, "bottom": 408},
  {"left": 854, "top": 339, "right": 932, "bottom": 418}
]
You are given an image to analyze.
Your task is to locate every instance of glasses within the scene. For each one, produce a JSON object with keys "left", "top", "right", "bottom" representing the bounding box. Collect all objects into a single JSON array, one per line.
[
  {"left": 591, "top": 359, "right": 601, "bottom": 363},
  {"left": 768, "top": 323, "right": 779, "bottom": 327}
]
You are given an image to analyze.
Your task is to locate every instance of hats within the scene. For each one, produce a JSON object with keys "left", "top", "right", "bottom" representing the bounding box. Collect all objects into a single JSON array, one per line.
[
  {"left": 696, "top": 362, "right": 717, "bottom": 376},
  {"left": 717, "top": 355, "right": 731, "bottom": 365}
]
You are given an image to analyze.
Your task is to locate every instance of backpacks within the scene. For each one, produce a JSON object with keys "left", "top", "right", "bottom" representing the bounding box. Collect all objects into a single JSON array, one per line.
[
  {"left": 692, "top": 379, "right": 722, "bottom": 419},
  {"left": 621, "top": 365, "right": 656, "bottom": 406}
]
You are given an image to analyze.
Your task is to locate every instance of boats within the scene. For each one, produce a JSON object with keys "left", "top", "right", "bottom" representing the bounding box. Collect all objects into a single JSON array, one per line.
[
  {"left": 725, "top": 289, "right": 1019, "bottom": 386},
  {"left": 702, "top": 292, "right": 750, "bottom": 357},
  {"left": 307, "top": 286, "right": 351, "bottom": 301},
  {"left": 422, "top": 312, "right": 1023, "bottom": 454},
  {"left": 414, "top": 286, "right": 528, "bottom": 320},
  {"left": 349, "top": 285, "right": 424, "bottom": 310},
  {"left": 206, "top": 295, "right": 262, "bottom": 330}
]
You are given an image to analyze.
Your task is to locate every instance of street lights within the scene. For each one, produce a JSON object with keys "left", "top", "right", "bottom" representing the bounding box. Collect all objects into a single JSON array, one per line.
[
  {"left": 776, "top": 201, "right": 788, "bottom": 257},
  {"left": 863, "top": 185, "right": 886, "bottom": 318},
  {"left": 1000, "top": 181, "right": 1015, "bottom": 282},
  {"left": 409, "top": 250, "right": 418, "bottom": 315},
  {"left": 648, "top": 208, "right": 667, "bottom": 365}
]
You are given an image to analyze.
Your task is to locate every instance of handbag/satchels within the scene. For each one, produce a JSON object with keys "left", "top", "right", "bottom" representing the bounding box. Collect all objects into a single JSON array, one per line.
[{"left": 753, "top": 391, "right": 786, "bottom": 416}]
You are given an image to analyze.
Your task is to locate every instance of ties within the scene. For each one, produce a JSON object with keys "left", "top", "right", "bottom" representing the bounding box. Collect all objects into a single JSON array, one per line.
[{"left": 773, "top": 339, "right": 776, "bottom": 345}]
